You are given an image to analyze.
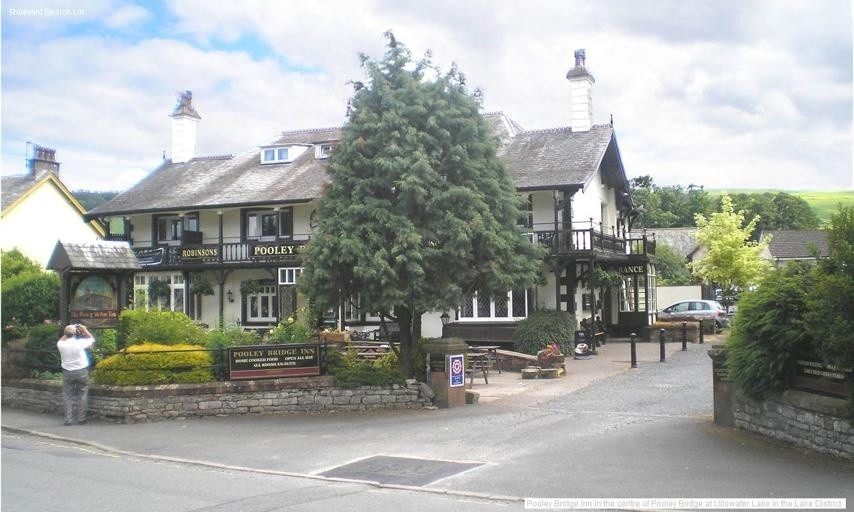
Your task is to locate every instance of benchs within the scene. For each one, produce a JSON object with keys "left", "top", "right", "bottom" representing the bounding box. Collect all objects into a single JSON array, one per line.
[
  {"left": 581, "top": 317, "right": 607, "bottom": 349},
  {"left": 343, "top": 341, "right": 401, "bottom": 365}
]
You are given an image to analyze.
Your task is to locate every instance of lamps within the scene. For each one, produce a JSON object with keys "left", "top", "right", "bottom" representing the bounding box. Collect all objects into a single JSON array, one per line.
[{"left": 226, "top": 289, "right": 233, "bottom": 303}]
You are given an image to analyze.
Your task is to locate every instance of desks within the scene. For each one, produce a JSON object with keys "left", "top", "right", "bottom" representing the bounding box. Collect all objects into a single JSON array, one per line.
[
  {"left": 467, "top": 345, "right": 502, "bottom": 374},
  {"left": 466, "top": 353, "right": 488, "bottom": 389}
]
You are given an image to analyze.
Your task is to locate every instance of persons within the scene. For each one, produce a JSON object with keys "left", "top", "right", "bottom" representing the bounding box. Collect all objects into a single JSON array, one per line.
[{"left": 56, "top": 323, "right": 96, "bottom": 426}]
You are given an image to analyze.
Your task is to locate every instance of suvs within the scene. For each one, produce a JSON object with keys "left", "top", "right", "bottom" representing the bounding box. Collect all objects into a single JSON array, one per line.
[{"left": 658, "top": 300, "right": 729, "bottom": 334}]
